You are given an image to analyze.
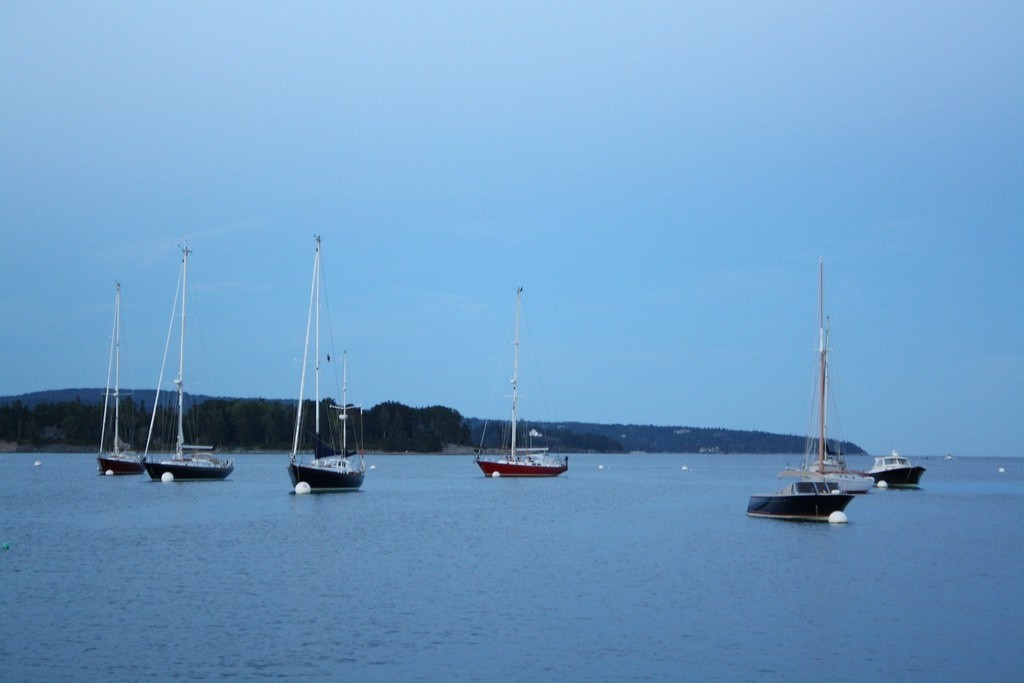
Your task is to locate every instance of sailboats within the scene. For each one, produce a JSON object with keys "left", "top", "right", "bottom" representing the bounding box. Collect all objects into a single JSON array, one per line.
[
  {"left": 743, "top": 256, "right": 857, "bottom": 523},
  {"left": 313, "top": 348, "right": 358, "bottom": 465},
  {"left": 471, "top": 285, "right": 569, "bottom": 479},
  {"left": 284, "top": 233, "right": 366, "bottom": 495},
  {"left": 798, "top": 311, "right": 876, "bottom": 494},
  {"left": 96, "top": 282, "right": 146, "bottom": 475},
  {"left": 143, "top": 239, "right": 235, "bottom": 482}
]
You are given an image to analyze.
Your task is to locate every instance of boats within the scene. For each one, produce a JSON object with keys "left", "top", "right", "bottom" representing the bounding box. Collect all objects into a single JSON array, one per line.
[{"left": 863, "top": 450, "right": 927, "bottom": 489}]
